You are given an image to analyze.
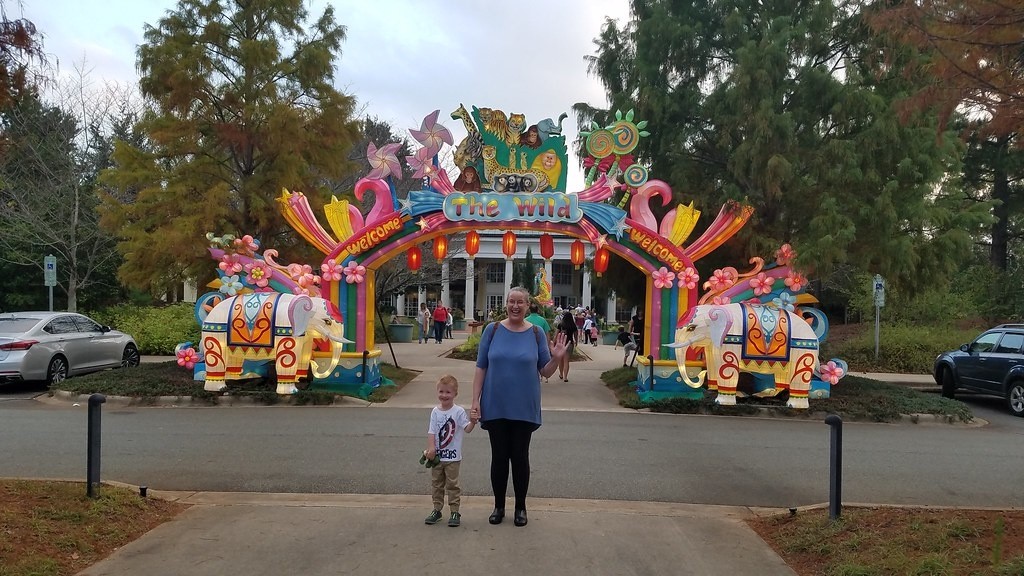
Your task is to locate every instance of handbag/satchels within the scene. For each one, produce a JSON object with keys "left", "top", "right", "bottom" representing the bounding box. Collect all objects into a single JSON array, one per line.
[{"left": 583, "top": 326, "right": 586, "bottom": 330}]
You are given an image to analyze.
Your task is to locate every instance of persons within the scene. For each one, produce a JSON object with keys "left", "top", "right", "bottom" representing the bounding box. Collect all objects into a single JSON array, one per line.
[
  {"left": 446, "top": 308, "right": 454, "bottom": 341},
  {"left": 524, "top": 304, "right": 578, "bottom": 383},
  {"left": 416, "top": 302, "right": 431, "bottom": 344},
  {"left": 615, "top": 305, "right": 644, "bottom": 369},
  {"left": 433, "top": 300, "right": 448, "bottom": 344},
  {"left": 471, "top": 286, "right": 571, "bottom": 527},
  {"left": 419, "top": 375, "right": 477, "bottom": 527},
  {"left": 554, "top": 303, "right": 598, "bottom": 347}
]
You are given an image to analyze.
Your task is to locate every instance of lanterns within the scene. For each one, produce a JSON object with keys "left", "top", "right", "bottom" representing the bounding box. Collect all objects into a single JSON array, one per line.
[
  {"left": 570, "top": 240, "right": 585, "bottom": 270},
  {"left": 594, "top": 249, "right": 609, "bottom": 278},
  {"left": 501, "top": 231, "right": 517, "bottom": 260},
  {"left": 407, "top": 247, "right": 422, "bottom": 274},
  {"left": 466, "top": 230, "right": 480, "bottom": 259},
  {"left": 432, "top": 236, "right": 449, "bottom": 265},
  {"left": 540, "top": 233, "right": 554, "bottom": 261}
]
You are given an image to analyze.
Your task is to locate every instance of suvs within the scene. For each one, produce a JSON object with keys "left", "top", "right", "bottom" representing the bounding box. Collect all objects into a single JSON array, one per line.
[{"left": 933, "top": 323, "right": 1024, "bottom": 418}]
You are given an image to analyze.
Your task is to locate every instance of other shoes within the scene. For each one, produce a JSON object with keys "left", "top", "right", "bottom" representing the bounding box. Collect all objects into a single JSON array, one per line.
[
  {"left": 425, "top": 340, "right": 428, "bottom": 343},
  {"left": 439, "top": 341, "right": 442, "bottom": 344},
  {"left": 419, "top": 341, "right": 421, "bottom": 343},
  {"left": 435, "top": 340, "right": 438, "bottom": 343},
  {"left": 623, "top": 364, "right": 628, "bottom": 367},
  {"left": 563, "top": 380, "right": 568, "bottom": 382},
  {"left": 447, "top": 336, "right": 449, "bottom": 339},
  {"left": 451, "top": 337, "right": 453, "bottom": 339},
  {"left": 559, "top": 375, "right": 563, "bottom": 379},
  {"left": 545, "top": 380, "right": 548, "bottom": 383},
  {"left": 540, "top": 377, "right": 543, "bottom": 380}
]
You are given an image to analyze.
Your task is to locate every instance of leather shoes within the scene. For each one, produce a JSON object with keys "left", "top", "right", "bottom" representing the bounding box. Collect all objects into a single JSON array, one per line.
[
  {"left": 514, "top": 509, "right": 527, "bottom": 527},
  {"left": 489, "top": 503, "right": 505, "bottom": 524}
]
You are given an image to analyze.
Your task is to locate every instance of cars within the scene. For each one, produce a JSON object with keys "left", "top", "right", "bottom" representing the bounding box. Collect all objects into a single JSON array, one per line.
[{"left": 0, "top": 310, "right": 141, "bottom": 391}]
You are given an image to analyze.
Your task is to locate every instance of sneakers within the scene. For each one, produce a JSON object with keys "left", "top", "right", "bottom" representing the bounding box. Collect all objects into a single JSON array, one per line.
[
  {"left": 447, "top": 512, "right": 461, "bottom": 526},
  {"left": 425, "top": 510, "right": 442, "bottom": 524}
]
